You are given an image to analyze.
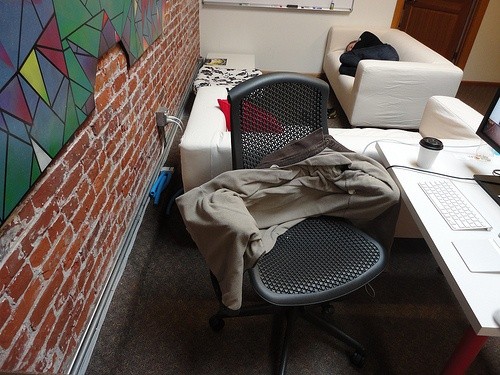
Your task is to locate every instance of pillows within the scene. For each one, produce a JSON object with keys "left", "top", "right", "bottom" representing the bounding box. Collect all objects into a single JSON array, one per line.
[{"left": 216, "top": 94, "right": 284, "bottom": 134}]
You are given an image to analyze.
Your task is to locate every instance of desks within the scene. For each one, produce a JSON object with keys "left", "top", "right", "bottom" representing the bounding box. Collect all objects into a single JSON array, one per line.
[{"left": 375, "top": 139, "right": 500, "bottom": 375}]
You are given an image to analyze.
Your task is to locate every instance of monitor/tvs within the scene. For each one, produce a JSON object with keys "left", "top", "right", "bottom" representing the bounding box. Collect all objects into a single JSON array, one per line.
[{"left": 472, "top": 88, "right": 500, "bottom": 208}]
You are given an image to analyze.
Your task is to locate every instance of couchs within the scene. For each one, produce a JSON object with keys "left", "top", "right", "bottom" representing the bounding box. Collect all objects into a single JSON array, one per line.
[
  {"left": 323, "top": 25, "right": 464, "bottom": 132},
  {"left": 178, "top": 83, "right": 483, "bottom": 198}
]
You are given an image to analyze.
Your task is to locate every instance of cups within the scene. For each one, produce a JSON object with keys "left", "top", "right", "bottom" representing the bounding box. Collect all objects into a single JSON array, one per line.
[{"left": 416, "top": 137, "right": 444, "bottom": 170}]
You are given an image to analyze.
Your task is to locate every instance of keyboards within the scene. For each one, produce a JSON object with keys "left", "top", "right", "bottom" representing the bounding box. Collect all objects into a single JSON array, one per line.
[{"left": 418, "top": 177, "right": 493, "bottom": 231}]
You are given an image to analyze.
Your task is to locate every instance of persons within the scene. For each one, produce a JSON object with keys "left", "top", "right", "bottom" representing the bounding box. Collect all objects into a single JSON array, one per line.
[{"left": 338, "top": 31, "right": 399, "bottom": 77}]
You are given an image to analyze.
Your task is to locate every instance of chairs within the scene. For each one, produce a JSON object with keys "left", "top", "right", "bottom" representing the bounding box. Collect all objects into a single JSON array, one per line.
[{"left": 226, "top": 71, "right": 402, "bottom": 375}]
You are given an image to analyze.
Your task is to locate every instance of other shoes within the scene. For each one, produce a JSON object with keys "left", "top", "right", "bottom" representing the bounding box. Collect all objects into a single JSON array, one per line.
[{"left": 327, "top": 108, "right": 337, "bottom": 119}]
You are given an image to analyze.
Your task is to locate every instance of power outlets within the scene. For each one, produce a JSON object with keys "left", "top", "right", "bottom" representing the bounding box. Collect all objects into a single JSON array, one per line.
[{"left": 157, "top": 107, "right": 170, "bottom": 127}]
